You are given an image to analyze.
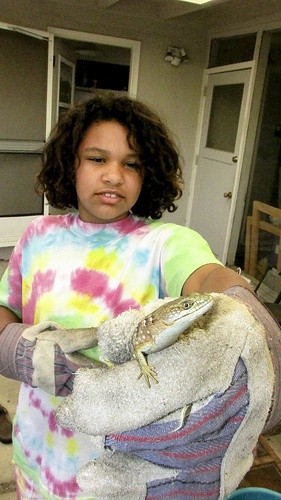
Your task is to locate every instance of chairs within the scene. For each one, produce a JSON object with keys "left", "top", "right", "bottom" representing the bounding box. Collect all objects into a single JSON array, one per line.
[{"left": 244, "top": 200, "right": 281, "bottom": 277}]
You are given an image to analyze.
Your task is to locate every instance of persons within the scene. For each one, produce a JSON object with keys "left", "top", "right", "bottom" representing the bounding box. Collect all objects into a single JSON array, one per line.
[{"left": 1, "top": 95, "right": 258, "bottom": 500}]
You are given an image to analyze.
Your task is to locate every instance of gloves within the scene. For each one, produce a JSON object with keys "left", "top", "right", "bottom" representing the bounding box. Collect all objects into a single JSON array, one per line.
[{"left": 0, "top": 323, "right": 107, "bottom": 397}]
